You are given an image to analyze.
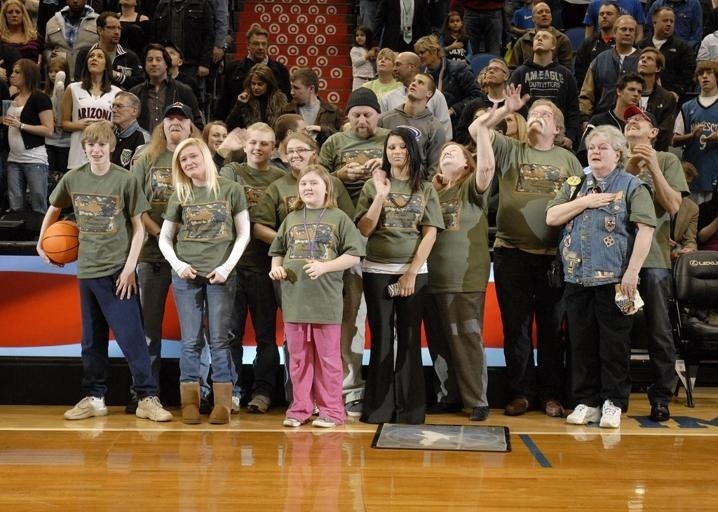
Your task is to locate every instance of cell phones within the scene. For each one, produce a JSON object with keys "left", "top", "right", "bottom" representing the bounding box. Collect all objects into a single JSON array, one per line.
[{"left": 387, "top": 282, "right": 399, "bottom": 297}]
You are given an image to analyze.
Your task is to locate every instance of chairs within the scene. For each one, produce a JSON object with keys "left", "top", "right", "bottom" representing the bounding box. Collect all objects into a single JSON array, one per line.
[
  {"left": 470, "top": 52, "right": 506, "bottom": 76},
  {"left": 669, "top": 247, "right": 717, "bottom": 410},
  {"left": 563, "top": 26, "right": 585, "bottom": 66}
]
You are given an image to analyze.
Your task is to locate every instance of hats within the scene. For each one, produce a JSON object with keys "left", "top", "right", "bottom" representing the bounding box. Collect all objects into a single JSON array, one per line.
[
  {"left": 165, "top": 102, "right": 193, "bottom": 122},
  {"left": 622, "top": 105, "right": 657, "bottom": 128},
  {"left": 345, "top": 87, "right": 382, "bottom": 115}
]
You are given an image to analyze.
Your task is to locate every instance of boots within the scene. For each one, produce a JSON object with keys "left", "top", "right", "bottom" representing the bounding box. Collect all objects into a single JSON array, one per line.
[
  {"left": 209, "top": 382, "right": 235, "bottom": 425},
  {"left": 179, "top": 380, "right": 201, "bottom": 425}
]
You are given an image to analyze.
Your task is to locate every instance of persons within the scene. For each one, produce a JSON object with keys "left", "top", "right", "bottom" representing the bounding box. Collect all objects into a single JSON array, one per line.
[
  {"left": 283, "top": 432, "right": 347, "bottom": 512},
  {"left": 0, "top": 0, "right": 718, "bottom": 427}
]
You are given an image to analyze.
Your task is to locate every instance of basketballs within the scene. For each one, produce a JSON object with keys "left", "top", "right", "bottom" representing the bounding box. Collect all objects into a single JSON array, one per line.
[{"left": 42, "top": 220, "right": 78, "bottom": 264}]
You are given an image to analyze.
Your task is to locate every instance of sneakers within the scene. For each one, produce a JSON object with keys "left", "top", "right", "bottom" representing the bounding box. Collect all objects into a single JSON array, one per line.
[
  {"left": 284, "top": 417, "right": 300, "bottom": 427},
  {"left": 345, "top": 399, "right": 362, "bottom": 417},
  {"left": 64, "top": 396, "right": 108, "bottom": 420},
  {"left": 600, "top": 400, "right": 622, "bottom": 427},
  {"left": 311, "top": 418, "right": 335, "bottom": 427},
  {"left": 651, "top": 404, "right": 670, "bottom": 421},
  {"left": 470, "top": 406, "right": 489, "bottom": 420},
  {"left": 505, "top": 398, "right": 528, "bottom": 416},
  {"left": 565, "top": 403, "right": 601, "bottom": 425},
  {"left": 546, "top": 400, "right": 566, "bottom": 417},
  {"left": 247, "top": 395, "right": 270, "bottom": 415},
  {"left": 135, "top": 396, "right": 173, "bottom": 422}
]
